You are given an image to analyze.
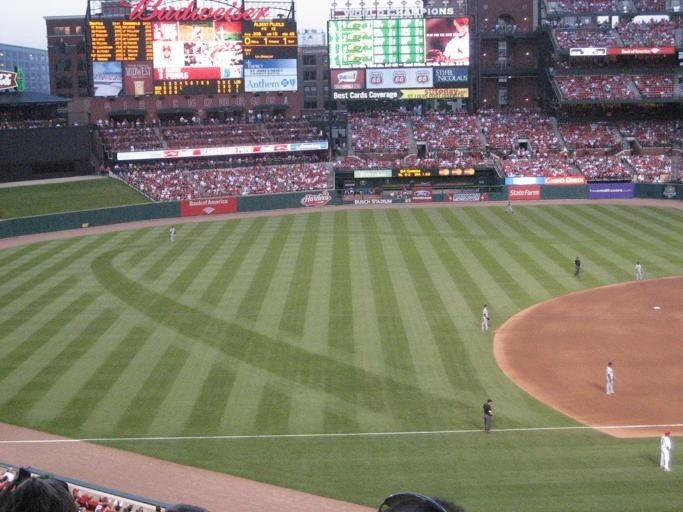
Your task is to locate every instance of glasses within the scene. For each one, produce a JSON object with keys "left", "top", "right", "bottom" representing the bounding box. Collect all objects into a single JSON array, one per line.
[{"left": 377, "top": 492, "right": 449, "bottom": 512}]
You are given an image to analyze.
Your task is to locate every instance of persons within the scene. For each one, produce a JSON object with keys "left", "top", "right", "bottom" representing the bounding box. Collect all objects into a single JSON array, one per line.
[
  {"left": 633, "top": 258, "right": 643, "bottom": 281},
  {"left": 481, "top": 303, "right": 489, "bottom": 333},
  {"left": 504, "top": 201, "right": 514, "bottom": 213},
  {"left": 541, "top": 0, "right": 683, "bottom": 100},
  {"left": 95, "top": 98, "right": 682, "bottom": 201},
  {"left": 573, "top": 256, "right": 582, "bottom": 276},
  {"left": 169, "top": 223, "right": 176, "bottom": 241},
  {"left": 483, "top": 20, "right": 531, "bottom": 34},
  {"left": 1, "top": 108, "right": 69, "bottom": 131},
  {"left": 428, "top": 18, "right": 469, "bottom": 66},
  {"left": 0, "top": 465, "right": 208, "bottom": 511},
  {"left": 605, "top": 360, "right": 615, "bottom": 395},
  {"left": 659, "top": 430, "right": 672, "bottom": 472},
  {"left": 482, "top": 398, "right": 495, "bottom": 433}
]
